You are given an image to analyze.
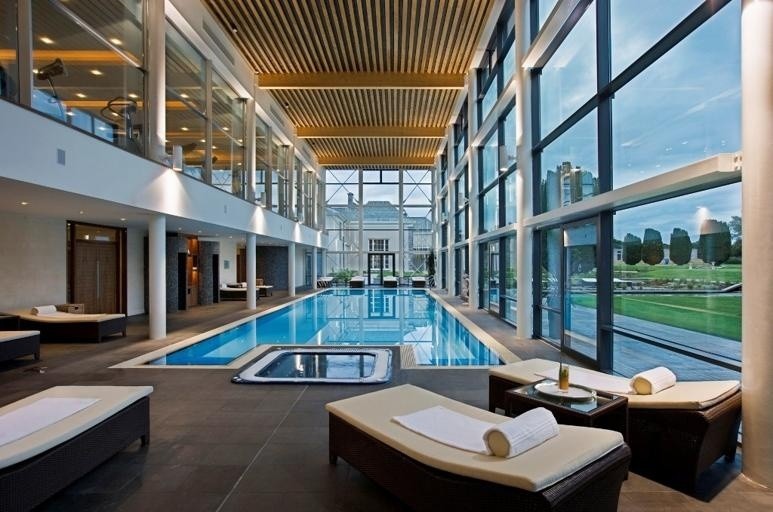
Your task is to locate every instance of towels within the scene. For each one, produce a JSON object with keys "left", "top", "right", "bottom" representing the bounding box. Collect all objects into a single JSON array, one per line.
[
  {"left": 392, "top": 405, "right": 559, "bottom": 459},
  {"left": 534, "top": 361, "right": 677, "bottom": 395},
  {"left": 0, "top": 397, "right": 101, "bottom": 446}
]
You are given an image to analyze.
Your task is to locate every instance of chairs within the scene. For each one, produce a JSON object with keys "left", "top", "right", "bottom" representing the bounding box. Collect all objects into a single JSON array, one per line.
[
  {"left": 0, "top": 305, "right": 129, "bottom": 363},
  {"left": 412, "top": 291, "right": 425, "bottom": 294},
  {"left": 350, "top": 290, "right": 364, "bottom": 295},
  {"left": 350, "top": 276, "right": 365, "bottom": 288},
  {"left": 324, "top": 382, "right": 633, "bottom": 512},
  {"left": 317, "top": 277, "right": 334, "bottom": 288},
  {"left": 412, "top": 277, "right": 426, "bottom": 287},
  {"left": 581, "top": 277, "right": 633, "bottom": 289},
  {"left": 0, "top": 385, "right": 154, "bottom": 512},
  {"left": 220, "top": 282, "right": 273, "bottom": 299},
  {"left": 488, "top": 357, "right": 742, "bottom": 491},
  {"left": 322, "top": 290, "right": 334, "bottom": 294},
  {"left": 384, "top": 276, "right": 397, "bottom": 288},
  {"left": 384, "top": 291, "right": 397, "bottom": 294}
]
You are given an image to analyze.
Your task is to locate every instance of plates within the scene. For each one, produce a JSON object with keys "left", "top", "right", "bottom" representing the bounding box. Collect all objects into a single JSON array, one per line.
[{"left": 533, "top": 382, "right": 597, "bottom": 399}]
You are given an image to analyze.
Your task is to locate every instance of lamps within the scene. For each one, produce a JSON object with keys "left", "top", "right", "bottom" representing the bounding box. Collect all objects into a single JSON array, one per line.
[{"left": 160, "top": 147, "right": 183, "bottom": 171}]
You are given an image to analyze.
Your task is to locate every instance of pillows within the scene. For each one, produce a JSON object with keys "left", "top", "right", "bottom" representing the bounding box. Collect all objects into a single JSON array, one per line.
[
  {"left": 634, "top": 377, "right": 652, "bottom": 394},
  {"left": 487, "top": 431, "right": 509, "bottom": 457}
]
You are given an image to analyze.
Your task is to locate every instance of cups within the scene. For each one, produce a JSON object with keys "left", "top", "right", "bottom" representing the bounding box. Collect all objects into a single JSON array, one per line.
[{"left": 559, "top": 363, "right": 569, "bottom": 393}]
